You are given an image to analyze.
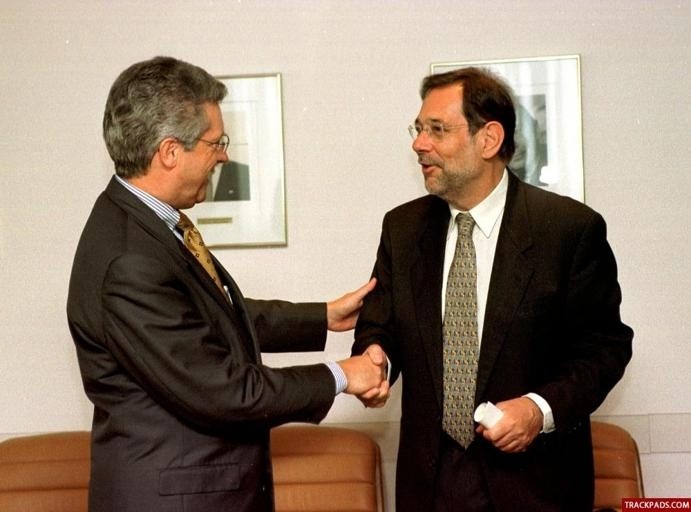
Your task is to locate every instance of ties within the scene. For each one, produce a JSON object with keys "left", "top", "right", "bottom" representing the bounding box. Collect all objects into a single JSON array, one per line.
[
  {"left": 441, "top": 214, "right": 480, "bottom": 451},
  {"left": 175, "top": 213, "right": 233, "bottom": 304}
]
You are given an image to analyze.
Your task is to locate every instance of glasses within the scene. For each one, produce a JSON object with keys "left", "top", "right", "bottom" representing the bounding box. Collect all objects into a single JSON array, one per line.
[
  {"left": 197, "top": 135, "right": 231, "bottom": 153},
  {"left": 407, "top": 125, "right": 472, "bottom": 140}
]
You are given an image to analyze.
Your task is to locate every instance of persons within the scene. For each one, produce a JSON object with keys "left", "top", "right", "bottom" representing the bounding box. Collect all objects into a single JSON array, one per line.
[
  {"left": 351, "top": 67, "right": 635, "bottom": 512},
  {"left": 65, "top": 55, "right": 388, "bottom": 512}
]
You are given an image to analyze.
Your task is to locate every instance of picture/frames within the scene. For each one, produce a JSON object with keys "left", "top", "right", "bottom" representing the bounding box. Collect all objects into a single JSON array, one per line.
[
  {"left": 179, "top": 71, "right": 289, "bottom": 251},
  {"left": 430, "top": 52, "right": 587, "bottom": 210}
]
